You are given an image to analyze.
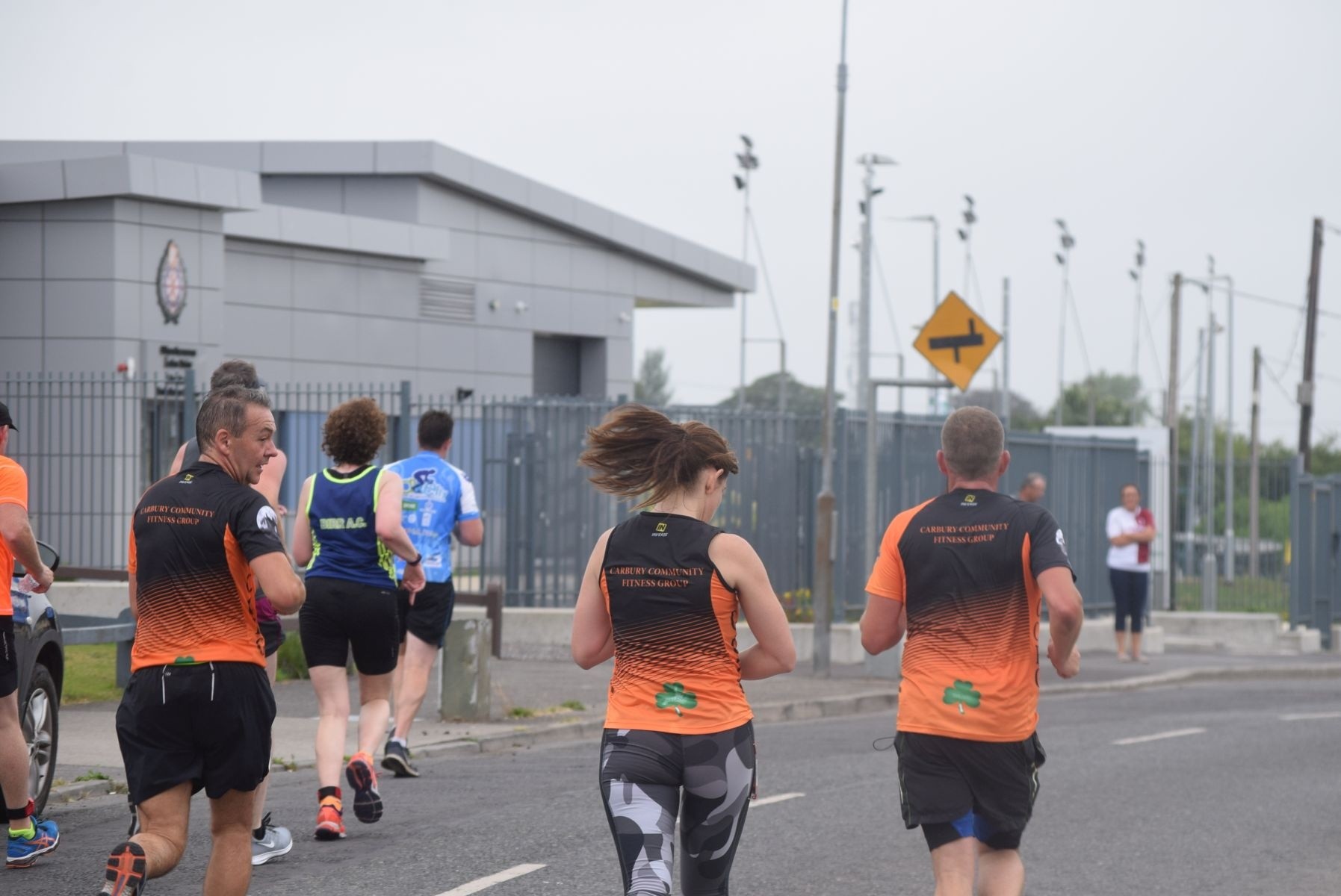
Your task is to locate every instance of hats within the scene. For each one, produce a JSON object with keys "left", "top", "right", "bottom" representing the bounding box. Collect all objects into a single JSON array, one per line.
[{"left": 0, "top": 402, "right": 18, "bottom": 431}]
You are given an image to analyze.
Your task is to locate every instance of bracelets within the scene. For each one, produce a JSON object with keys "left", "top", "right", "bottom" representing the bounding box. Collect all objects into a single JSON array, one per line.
[{"left": 406, "top": 553, "right": 422, "bottom": 567}]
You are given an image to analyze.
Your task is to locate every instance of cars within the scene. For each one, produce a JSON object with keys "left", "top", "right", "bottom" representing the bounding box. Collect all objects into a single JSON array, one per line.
[{"left": 0, "top": 539, "right": 64, "bottom": 826}]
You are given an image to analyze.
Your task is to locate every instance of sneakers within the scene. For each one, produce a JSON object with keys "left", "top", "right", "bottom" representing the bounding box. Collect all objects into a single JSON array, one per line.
[
  {"left": 381, "top": 741, "right": 419, "bottom": 777},
  {"left": 315, "top": 807, "right": 347, "bottom": 838},
  {"left": 346, "top": 752, "right": 384, "bottom": 824},
  {"left": 252, "top": 811, "right": 294, "bottom": 865},
  {"left": 6, "top": 815, "right": 61, "bottom": 868}
]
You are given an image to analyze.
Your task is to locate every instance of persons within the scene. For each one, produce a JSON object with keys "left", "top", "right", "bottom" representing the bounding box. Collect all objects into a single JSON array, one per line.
[
  {"left": 99, "top": 387, "right": 306, "bottom": 896},
  {"left": 0, "top": 401, "right": 60, "bottom": 869},
  {"left": 1106, "top": 482, "right": 1156, "bottom": 663},
  {"left": 570, "top": 401, "right": 794, "bottom": 896},
  {"left": 124, "top": 360, "right": 292, "bottom": 866},
  {"left": 382, "top": 408, "right": 484, "bottom": 778},
  {"left": 858, "top": 406, "right": 1084, "bottom": 896},
  {"left": 1008, "top": 471, "right": 1048, "bottom": 506},
  {"left": 291, "top": 400, "right": 427, "bottom": 841}
]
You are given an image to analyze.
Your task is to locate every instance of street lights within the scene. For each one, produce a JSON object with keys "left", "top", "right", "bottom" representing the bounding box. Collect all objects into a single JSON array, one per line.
[
  {"left": 1169, "top": 272, "right": 1212, "bottom": 609},
  {"left": 906, "top": 214, "right": 941, "bottom": 414}
]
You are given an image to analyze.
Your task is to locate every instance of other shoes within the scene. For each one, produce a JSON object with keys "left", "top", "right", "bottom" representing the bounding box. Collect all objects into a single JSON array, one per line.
[{"left": 97, "top": 842, "right": 147, "bottom": 896}]
